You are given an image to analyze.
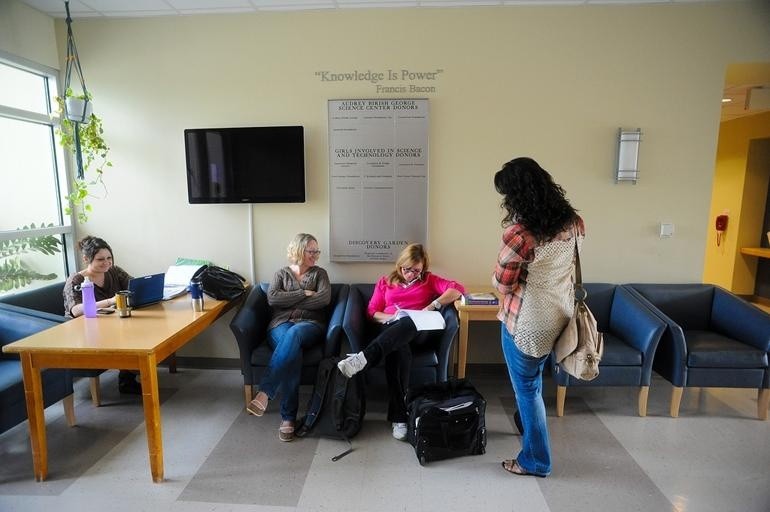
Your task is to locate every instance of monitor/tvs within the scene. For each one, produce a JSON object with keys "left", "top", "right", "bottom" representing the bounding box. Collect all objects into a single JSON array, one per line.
[{"left": 183, "top": 125, "right": 307, "bottom": 205}]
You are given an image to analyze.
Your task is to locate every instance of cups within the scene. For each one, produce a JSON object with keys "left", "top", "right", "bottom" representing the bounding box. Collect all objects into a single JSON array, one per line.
[{"left": 115, "top": 290, "right": 132, "bottom": 317}]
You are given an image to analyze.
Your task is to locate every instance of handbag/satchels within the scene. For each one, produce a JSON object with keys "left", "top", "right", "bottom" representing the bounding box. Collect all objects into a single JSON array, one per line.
[
  {"left": 192, "top": 265, "right": 247, "bottom": 301},
  {"left": 554, "top": 299, "right": 604, "bottom": 382},
  {"left": 405, "top": 377, "right": 487, "bottom": 465}
]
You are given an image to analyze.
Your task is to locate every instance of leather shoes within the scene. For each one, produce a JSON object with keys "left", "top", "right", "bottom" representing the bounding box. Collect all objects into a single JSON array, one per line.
[{"left": 120, "top": 383, "right": 142, "bottom": 395}]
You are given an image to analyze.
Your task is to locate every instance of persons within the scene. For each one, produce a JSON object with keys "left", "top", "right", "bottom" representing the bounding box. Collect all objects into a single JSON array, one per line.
[
  {"left": 338, "top": 242, "right": 466, "bottom": 440},
  {"left": 245, "top": 232, "right": 332, "bottom": 440},
  {"left": 490, "top": 156, "right": 583, "bottom": 479},
  {"left": 62, "top": 234, "right": 142, "bottom": 398}
]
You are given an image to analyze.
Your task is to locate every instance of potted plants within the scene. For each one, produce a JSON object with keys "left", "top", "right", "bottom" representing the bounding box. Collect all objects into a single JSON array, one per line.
[{"left": 51, "top": 87, "right": 114, "bottom": 226}]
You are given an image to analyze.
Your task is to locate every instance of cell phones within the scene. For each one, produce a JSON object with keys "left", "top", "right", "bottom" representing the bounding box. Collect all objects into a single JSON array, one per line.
[{"left": 96, "top": 309, "right": 115, "bottom": 314}]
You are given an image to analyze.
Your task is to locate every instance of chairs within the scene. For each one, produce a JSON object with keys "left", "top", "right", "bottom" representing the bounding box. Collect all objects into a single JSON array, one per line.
[
  {"left": 1, "top": 281, "right": 179, "bottom": 408},
  {"left": 621, "top": 284, "right": 770, "bottom": 420},
  {"left": 229, "top": 283, "right": 348, "bottom": 413},
  {"left": 0, "top": 309, "right": 77, "bottom": 435},
  {"left": 341, "top": 283, "right": 461, "bottom": 403},
  {"left": 541, "top": 284, "right": 668, "bottom": 417}
]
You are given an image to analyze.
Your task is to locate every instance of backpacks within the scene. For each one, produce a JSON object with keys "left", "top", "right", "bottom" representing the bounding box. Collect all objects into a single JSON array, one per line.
[{"left": 295, "top": 356, "right": 366, "bottom": 440}]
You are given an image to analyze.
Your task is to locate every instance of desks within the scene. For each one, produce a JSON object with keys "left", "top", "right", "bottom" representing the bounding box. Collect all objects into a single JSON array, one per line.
[
  {"left": 453, "top": 283, "right": 503, "bottom": 379},
  {"left": 1, "top": 282, "right": 254, "bottom": 483}
]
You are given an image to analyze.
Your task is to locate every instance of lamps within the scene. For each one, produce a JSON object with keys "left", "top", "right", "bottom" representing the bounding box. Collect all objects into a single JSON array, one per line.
[{"left": 614, "top": 127, "right": 644, "bottom": 185}]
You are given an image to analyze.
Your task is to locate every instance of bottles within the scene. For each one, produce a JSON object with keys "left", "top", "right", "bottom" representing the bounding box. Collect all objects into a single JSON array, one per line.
[
  {"left": 191, "top": 278, "right": 205, "bottom": 312},
  {"left": 81, "top": 275, "right": 96, "bottom": 317}
]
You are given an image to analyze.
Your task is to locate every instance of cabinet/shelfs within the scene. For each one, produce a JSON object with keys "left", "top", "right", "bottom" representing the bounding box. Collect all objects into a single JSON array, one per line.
[{"left": 703, "top": 63, "right": 770, "bottom": 316}]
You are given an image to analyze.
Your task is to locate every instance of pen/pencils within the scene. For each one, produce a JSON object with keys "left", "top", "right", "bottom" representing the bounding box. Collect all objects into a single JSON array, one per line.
[{"left": 393, "top": 302, "right": 400, "bottom": 310}]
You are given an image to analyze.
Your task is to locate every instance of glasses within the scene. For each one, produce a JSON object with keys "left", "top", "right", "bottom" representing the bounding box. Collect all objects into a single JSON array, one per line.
[
  {"left": 305, "top": 250, "right": 321, "bottom": 255},
  {"left": 403, "top": 267, "right": 421, "bottom": 274}
]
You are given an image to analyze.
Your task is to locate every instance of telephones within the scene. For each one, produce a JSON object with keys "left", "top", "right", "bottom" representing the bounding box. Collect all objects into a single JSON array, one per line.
[{"left": 715, "top": 216, "right": 729, "bottom": 231}]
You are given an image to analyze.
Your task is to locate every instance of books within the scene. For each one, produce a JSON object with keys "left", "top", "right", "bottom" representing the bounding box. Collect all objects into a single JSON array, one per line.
[
  {"left": 161, "top": 264, "right": 203, "bottom": 301},
  {"left": 383, "top": 308, "right": 448, "bottom": 332},
  {"left": 461, "top": 292, "right": 499, "bottom": 305}
]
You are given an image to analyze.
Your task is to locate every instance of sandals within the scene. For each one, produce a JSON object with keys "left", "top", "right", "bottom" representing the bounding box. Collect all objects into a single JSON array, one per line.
[
  {"left": 502, "top": 459, "right": 546, "bottom": 478},
  {"left": 514, "top": 410, "right": 524, "bottom": 434}
]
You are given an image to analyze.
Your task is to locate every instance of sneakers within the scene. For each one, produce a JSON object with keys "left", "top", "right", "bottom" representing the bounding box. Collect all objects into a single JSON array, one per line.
[
  {"left": 391, "top": 421, "right": 409, "bottom": 441},
  {"left": 247, "top": 399, "right": 268, "bottom": 417},
  {"left": 279, "top": 420, "right": 295, "bottom": 441},
  {"left": 338, "top": 351, "right": 368, "bottom": 378}
]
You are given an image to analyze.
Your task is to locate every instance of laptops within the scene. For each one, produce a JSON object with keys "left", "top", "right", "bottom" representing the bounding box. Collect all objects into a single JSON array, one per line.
[{"left": 114, "top": 272, "right": 166, "bottom": 309}]
